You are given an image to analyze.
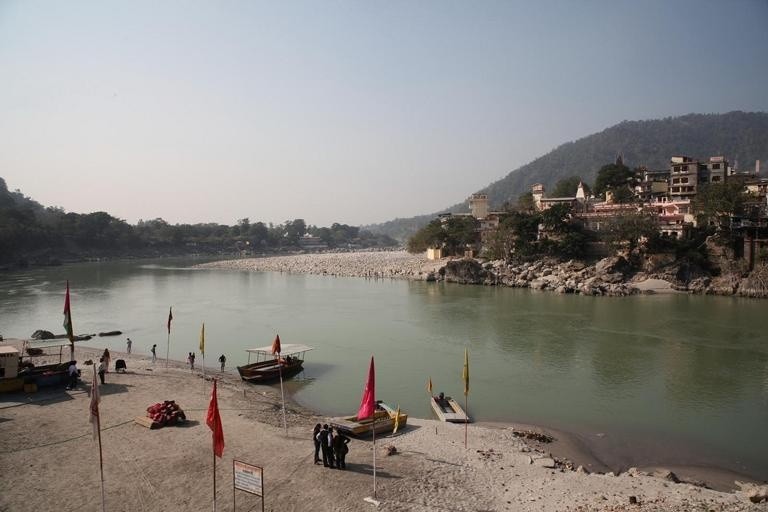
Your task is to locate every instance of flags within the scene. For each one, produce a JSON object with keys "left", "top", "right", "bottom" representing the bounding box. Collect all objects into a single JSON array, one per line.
[
  {"left": 167, "top": 308, "right": 172, "bottom": 335},
  {"left": 357, "top": 355, "right": 376, "bottom": 420},
  {"left": 87, "top": 363, "right": 103, "bottom": 442},
  {"left": 462, "top": 347, "right": 470, "bottom": 396},
  {"left": 62, "top": 283, "right": 75, "bottom": 343},
  {"left": 205, "top": 380, "right": 226, "bottom": 458},
  {"left": 271, "top": 335, "right": 281, "bottom": 354},
  {"left": 427, "top": 378, "right": 433, "bottom": 393},
  {"left": 199, "top": 323, "right": 205, "bottom": 359},
  {"left": 392, "top": 406, "right": 400, "bottom": 434}
]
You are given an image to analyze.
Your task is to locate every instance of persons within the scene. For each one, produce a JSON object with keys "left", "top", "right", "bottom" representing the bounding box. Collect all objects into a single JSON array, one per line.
[
  {"left": 281, "top": 355, "right": 297, "bottom": 367},
  {"left": 150, "top": 344, "right": 157, "bottom": 360},
  {"left": 126, "top": 338, "right": 132, "bottom": 354},
  {"left": 20, "top": 344, "right": 111, "bottom": 391},
  {"left": 187, "top": 352, "right": 195, "bottom": 371},
  {"left": 312, "top": 423, "right": 351, "bottom": 470},
  {"left": 219, "top": 354, "right": 226, "bottom": 373}
]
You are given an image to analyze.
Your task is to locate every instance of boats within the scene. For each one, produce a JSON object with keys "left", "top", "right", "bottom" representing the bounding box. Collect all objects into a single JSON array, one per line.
[
  {"left": 430, "top": 392, "right": 470, "bottom": 423},
  {"left": 329, "top": 401, "right": 407, "bottom": 438},
  {"left": 237, "top": 344, "right": 312, "bottom": 385}
]
